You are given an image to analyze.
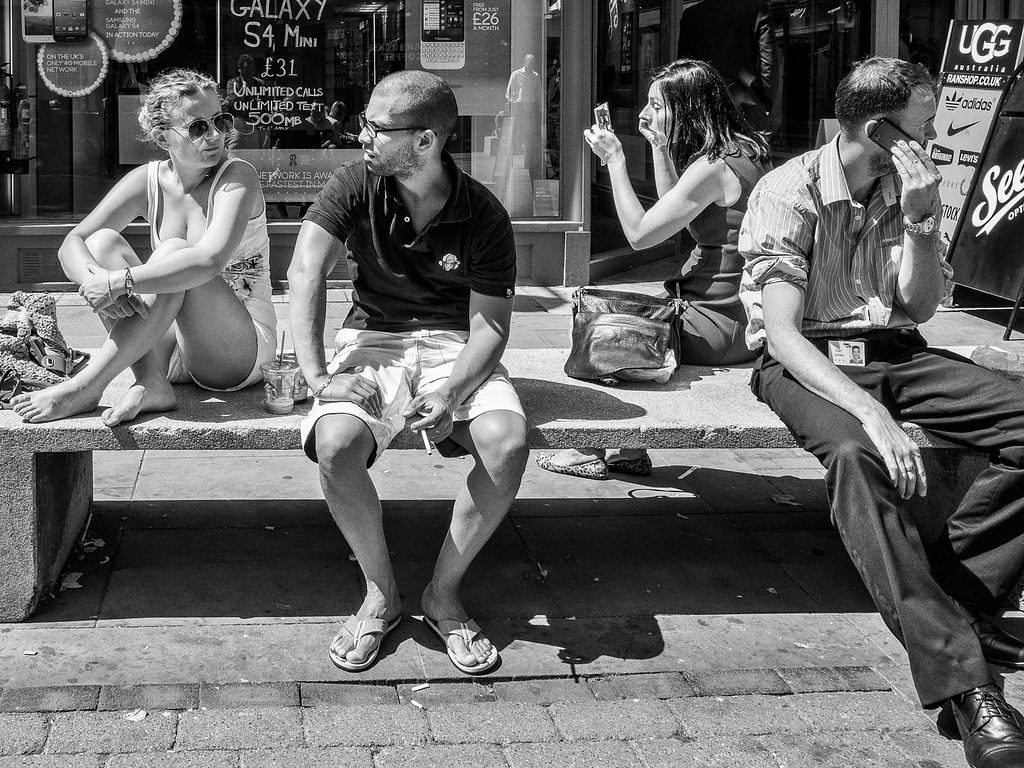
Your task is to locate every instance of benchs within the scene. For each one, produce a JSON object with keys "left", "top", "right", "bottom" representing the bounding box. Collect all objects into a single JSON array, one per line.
[{"left": 0, "top": 346, "right": 1009, "bottom": 623}]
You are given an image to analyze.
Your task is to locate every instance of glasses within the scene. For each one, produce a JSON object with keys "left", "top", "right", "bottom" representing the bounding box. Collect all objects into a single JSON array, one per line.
[
  {"left": 357, "top": 111, "right": 437, "bottom": 139},
  {"left": 167, "top": 112, "right": 235, "bottom": 142}
]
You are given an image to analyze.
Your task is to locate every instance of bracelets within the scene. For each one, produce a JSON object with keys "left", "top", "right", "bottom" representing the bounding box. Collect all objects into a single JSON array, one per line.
[
  {"left": 313, "top": 374, "right": 333, "bottom": 398},
  {"left": 107, "top": 272, "right": 115, "bottom": 306}
]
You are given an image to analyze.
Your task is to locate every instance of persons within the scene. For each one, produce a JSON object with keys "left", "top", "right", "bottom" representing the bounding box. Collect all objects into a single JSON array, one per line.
[
  {"left": 545, "top": 58, "right": 561, "bottom": 178},
  {"left": 737, "top": 58, "right": 1024, "bottom": 768},
  {"left": 222, "top": 56, "right": 365, "bottom": 219},
  {"left": 849, "top": 346, "right": 864, "bottom": 364},
  {"left": 287, "top": 72, "right": 530, "bottom": 674},
  {"left": 10, "top": 69, "right": 278, "bottom": 428},
  {"left": 507, "top": 55, "right": 542, "bottom": 178},
  {"left": 677, "top": 0, "right": 783, "bottom": 145},
  {"left": 535, "top": 60, "right": 774, "bottom": 481}
]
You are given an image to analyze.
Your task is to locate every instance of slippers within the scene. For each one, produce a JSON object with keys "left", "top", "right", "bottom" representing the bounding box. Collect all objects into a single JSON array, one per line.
[
  {"left": 328, "top": 604, "right": 402, "bottom": 673},
  {"left": 423, "top": 614, "right": 499, "bottom": 674}
]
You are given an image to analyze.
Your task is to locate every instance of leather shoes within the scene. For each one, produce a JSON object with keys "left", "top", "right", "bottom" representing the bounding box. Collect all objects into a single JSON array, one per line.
[
  {"left": 970, "top": 616, "right": 1024, "bottom": 669},
  {"left": 950, "top": 683, "right": 1024, "bottom": 768}
]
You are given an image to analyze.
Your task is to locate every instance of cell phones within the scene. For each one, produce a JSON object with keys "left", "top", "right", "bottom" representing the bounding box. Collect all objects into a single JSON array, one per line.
[{"left": 868, "top": 117, "right": 925, "bottom": 158}]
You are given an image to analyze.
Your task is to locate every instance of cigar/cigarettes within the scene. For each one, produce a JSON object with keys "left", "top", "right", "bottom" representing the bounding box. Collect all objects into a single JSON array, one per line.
[{"left": 420, "top": 429, "right": 431, "bottom": 456}]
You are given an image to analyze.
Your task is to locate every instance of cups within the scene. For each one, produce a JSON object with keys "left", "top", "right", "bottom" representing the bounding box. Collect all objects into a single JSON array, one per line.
[
  {"left": 258, "top": 360, "right": 301, "bottom": 416},
  {"left": 277, "top": 353, "right": 309, "bottom": 405}
]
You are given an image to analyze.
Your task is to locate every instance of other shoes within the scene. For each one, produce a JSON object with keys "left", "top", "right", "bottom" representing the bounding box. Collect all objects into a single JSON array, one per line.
[
  {"left": 536, "top": 451, "right": 609, "bottom": 480},
  {"left": 607, "top": 454, "right": 653, "bottom": 477}
]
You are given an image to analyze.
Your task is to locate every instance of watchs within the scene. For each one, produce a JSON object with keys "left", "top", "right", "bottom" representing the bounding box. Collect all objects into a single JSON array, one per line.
[
  {"left": 902, "top": 214, "right": 936, "bottom": 236},
  {"left": 124, "top": 267, "right": 134, "bottom": 298}
]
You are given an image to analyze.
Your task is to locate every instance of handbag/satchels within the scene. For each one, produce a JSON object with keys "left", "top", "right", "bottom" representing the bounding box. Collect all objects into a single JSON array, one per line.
[
  {"left": 565, "top": 287, "right": 685, "bottom": 386},
  {"left": 0, "top": 291, "right": 91, "bottom": 410}
]
